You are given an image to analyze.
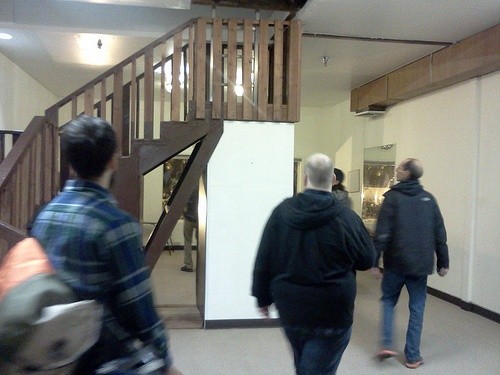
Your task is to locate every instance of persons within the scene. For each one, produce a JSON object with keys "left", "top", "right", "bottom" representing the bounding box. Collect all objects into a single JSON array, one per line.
[
  {"left": 250, "top": 153, "right": 377, "bottom": 375},
  {"left": 332, "top": 168, "right": 350, "bottom": 208},
  {"left": 372, "top": 158, "right": 450, "bottom": 368},
  {"left": 180, "top": 186, "right": 199, "bottom": 273},
  {"left": 30, "top": 114, "right": 183, "bottom": 375}
]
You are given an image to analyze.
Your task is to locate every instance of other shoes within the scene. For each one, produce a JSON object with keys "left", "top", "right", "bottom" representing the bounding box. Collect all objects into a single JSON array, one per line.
[
  {"left": 376, "top": 348, "right": 398, "bottom": 360},
  {"left": 406, "top": 359, "right": 425, "bottom": 368}
]
